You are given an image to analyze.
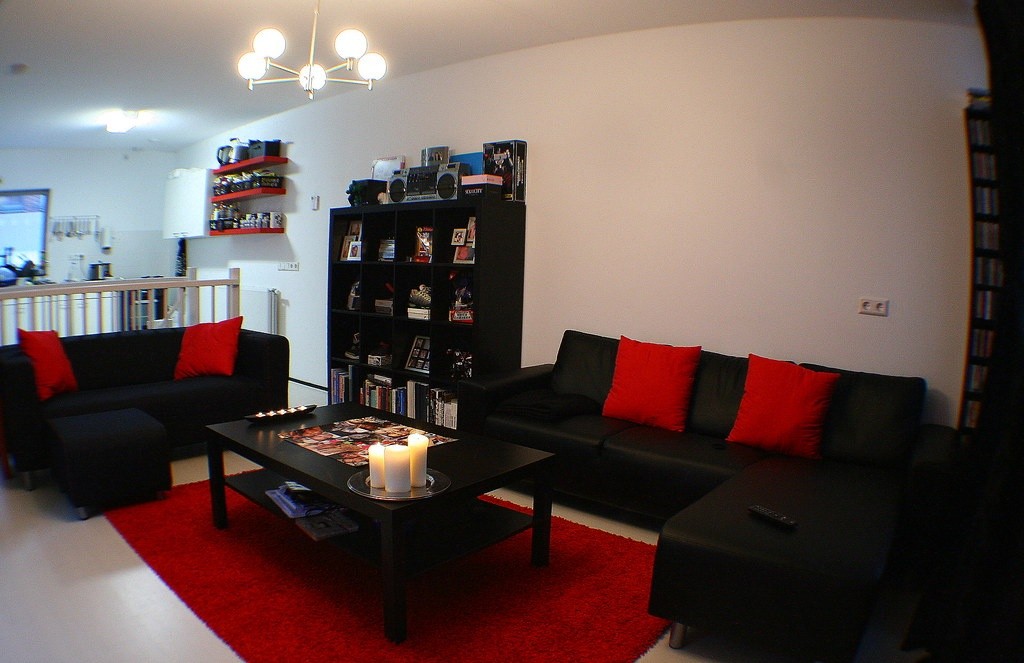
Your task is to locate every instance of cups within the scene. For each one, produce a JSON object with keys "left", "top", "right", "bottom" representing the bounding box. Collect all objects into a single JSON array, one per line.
[{"left": 240, "top": 212, "right": 282, "bottom": 228}]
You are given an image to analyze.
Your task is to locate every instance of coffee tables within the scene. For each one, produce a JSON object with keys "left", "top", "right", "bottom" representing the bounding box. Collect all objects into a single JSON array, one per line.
[{"left": 206, "top": 403, "right": 555, "bottom": 645}]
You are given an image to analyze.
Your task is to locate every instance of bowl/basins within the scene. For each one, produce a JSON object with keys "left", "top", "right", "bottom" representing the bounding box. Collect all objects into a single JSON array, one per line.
[{"left": 104, "top": 277, "right": 114, "bottom": 280}]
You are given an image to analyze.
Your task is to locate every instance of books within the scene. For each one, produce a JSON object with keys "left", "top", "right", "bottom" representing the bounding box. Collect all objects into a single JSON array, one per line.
[
  {"left": 265, "top": 482, "right": 359, "bottom": 542},
  {"left": 330, "top": 368, "right": 349, "bottom": 405},
  {"left": 360, "top": 374, "right": 429, "bottom": 422},
  {"left": 429, "top": 388, "right": 457, "bottom": 430}
]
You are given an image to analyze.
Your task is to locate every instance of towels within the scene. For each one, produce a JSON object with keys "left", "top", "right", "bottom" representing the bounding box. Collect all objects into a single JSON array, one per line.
[{"left": 174, "top": 238, "right": 186, "bottom": 292}]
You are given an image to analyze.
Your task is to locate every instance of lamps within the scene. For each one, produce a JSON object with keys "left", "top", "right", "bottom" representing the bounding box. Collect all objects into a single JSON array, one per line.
[{"left": 238, "top": 0, "right": 386, "bottom": 101}]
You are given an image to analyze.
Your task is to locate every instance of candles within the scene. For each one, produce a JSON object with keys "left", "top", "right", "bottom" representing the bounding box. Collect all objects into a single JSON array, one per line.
[
  {"left": 385, "top": 443, "right": 411, "bottom": 494},
  {"left": 407, "top": 434, "right": 428, "bottom": 488},
  {"left": 368, "top": 442, "right": 385, "bottom": 488}
]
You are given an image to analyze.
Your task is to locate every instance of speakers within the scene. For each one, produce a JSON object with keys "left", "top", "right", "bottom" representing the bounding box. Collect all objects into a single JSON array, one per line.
[
  {"left": 387, "top": 169, "right": 409, "bottom": 204},
  {"left": 435, "top": 162, "right": 471, "bottom": 201}
]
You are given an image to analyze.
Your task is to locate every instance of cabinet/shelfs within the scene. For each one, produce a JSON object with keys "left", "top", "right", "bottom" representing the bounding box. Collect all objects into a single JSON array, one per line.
[
  {"left": 210, "top": 156, "right": 288, "bottom": 237},
  {"left": 958, "top": 90, "right": 1003, "bottom": 450},
  {"left": 327, "top": 197, "right": 526, "bottom": 433}
]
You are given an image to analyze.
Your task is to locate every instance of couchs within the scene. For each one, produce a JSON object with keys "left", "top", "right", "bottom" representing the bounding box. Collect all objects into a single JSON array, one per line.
[
  {"left": 0, "top": 327, "right": 290, "bottom": 492},
  {"left": 456, "top": 330, "right": 956, "bottom": 663}
]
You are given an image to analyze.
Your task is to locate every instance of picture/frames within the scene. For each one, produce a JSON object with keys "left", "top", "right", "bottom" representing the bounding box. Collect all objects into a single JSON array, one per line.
[
  {"left": 340, "top": 219, "right": 361, "bottom": 262},
  {"left": 451, "top": 216, "right": 476, "bottom": 264},
  {"left": 371, "top": 155, "right": 405, "bottom": 181}
]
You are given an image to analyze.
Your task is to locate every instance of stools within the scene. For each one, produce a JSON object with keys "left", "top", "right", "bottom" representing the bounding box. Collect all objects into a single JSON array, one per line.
[{"left": 42, "top": 407, "right": 171, "bottom": 520}]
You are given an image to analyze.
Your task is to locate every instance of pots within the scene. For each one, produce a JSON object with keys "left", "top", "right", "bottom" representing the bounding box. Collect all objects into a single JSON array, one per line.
[{"left": 87, "top": 262, "right": 111, "bottom": 279}]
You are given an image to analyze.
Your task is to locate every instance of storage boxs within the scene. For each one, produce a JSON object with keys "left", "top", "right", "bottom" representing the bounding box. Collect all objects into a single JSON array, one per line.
[
  {"left": 461, "top": 174, "right": 502, "bottom": 199},
  {"left": 482, "top": 140, "right": 527, "bottom": 202},
  {"left": 248, "top": 141, "right": 280, "bottom": 159}
]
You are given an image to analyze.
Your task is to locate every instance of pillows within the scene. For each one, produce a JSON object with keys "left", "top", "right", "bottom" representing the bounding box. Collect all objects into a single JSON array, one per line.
[
  {"left": 17, "top": 327, "right": 79, "bottom": 403},
  {"left": 601, "top": 334, "right": 701, "bottom": 434},
  {"left": 725, "top": 354, "right": 842, "bottom": 461},
  {"left": 173, "top": 316, "right": 244, "bottom": 380}
]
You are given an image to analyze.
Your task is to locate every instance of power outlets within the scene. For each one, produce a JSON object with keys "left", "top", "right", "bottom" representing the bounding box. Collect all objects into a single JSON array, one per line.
[{"left": 858, "top": 299, "right": 889, "bottom": 317}]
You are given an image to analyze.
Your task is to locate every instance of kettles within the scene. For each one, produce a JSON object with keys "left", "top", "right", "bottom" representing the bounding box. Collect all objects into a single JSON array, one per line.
[{"left": 216, "top": 144, "right": 233, "bottom": 169}]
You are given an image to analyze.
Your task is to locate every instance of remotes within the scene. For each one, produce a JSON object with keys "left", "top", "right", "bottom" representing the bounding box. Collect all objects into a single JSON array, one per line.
[{"left": 748, "top": 506, "right": 798, "bottom": 526}]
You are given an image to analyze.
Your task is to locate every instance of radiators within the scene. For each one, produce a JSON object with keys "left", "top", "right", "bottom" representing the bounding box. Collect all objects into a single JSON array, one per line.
[{"left": 240, "top": 288, "right": 281, "bottom": 335}]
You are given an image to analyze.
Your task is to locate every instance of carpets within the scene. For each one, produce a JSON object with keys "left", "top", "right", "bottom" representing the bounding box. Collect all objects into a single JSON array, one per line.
[{"left": 104, "top": 469, "right": 674, "bottom": 663}]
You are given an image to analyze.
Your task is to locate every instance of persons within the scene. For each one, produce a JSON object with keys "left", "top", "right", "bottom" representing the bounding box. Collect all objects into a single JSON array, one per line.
[
  {"left": 454, "top": 220, "right": 476, "bottom": 260},
  {"left": 343, "top": 224, "right": 360, "bottom": 256},
  {"left": 428, "top": 151, "right": 442, "bottom": 161}
]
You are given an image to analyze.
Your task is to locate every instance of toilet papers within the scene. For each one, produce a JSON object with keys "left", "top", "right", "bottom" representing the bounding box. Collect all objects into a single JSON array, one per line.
[{"left": 100, "top": 225, "right": 111, "bottom": 249}]
